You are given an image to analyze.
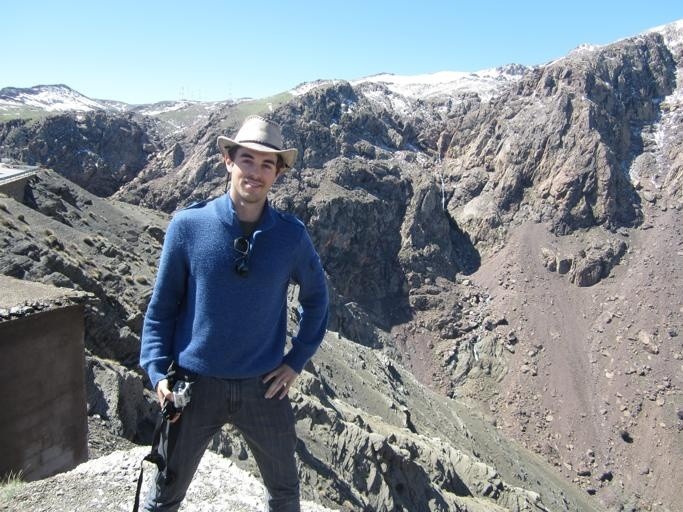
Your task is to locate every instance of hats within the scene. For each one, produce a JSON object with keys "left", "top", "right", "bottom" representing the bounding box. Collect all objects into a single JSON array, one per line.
[{"left": 217, "top": 114, "right": 298, "bottom": 177}]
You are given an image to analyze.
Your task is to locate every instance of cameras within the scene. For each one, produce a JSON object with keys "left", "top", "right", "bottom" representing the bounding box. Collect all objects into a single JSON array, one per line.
[{"left": 172, "top": 380, "right": 192, "bottom": 409}]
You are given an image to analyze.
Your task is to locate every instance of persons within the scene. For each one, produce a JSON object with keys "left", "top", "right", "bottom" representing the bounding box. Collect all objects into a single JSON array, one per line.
[{"left": 137, "top": 115, "right": 331, "bottom": 512}]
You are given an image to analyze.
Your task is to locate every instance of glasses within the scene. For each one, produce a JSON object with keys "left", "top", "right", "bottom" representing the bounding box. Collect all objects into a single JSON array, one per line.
[{"left": 234, "top": 236, "right": 250, "bottom": 278}]
